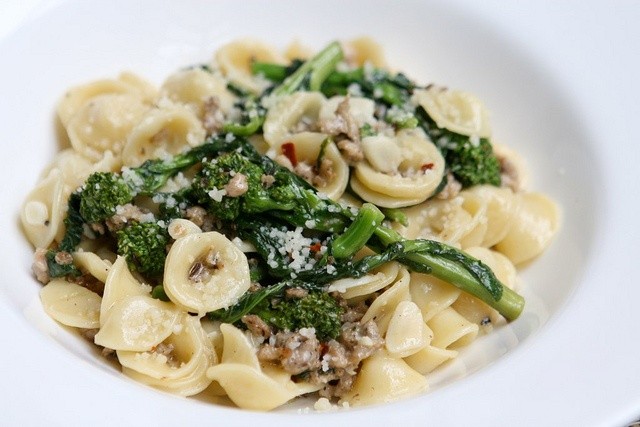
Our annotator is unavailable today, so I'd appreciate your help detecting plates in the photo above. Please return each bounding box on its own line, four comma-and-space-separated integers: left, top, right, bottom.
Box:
0, 1, 637, 427
